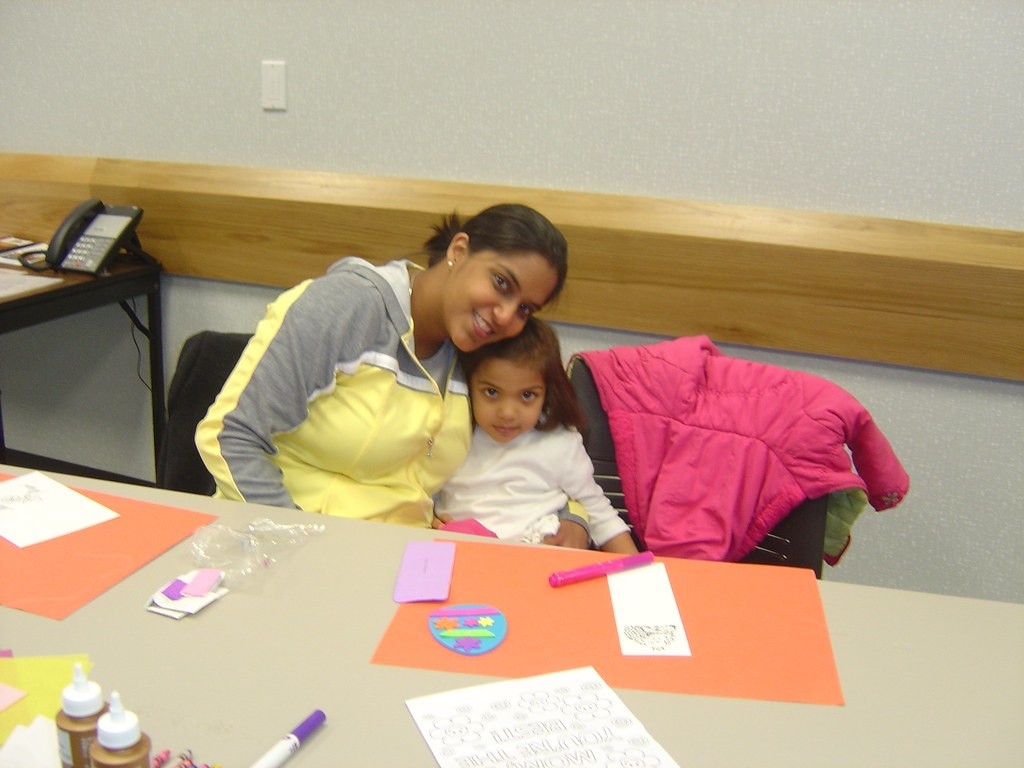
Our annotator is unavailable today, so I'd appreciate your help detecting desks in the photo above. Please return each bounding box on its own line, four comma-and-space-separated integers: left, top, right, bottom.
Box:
1, 226, 170, 486
1, 463, 1024, 765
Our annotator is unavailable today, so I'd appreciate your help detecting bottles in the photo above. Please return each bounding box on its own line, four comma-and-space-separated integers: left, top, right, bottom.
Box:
88, 692, 154, 767
53, 663, 119, 767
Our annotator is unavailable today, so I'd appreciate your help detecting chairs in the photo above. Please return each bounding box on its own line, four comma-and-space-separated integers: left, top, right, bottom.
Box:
558, 348, 833, 585
158, 327, 265, 496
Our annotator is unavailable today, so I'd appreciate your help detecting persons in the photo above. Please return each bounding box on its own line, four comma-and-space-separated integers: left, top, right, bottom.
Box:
423, 314, 643, 559
194, 203, 592, 558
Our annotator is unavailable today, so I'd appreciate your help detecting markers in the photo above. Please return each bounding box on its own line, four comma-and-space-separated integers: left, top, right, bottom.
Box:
548, 551, 656, 590
254, 708, 326, 767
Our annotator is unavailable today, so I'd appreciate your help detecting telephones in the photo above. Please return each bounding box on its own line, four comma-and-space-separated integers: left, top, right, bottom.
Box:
44, 197, 144, 278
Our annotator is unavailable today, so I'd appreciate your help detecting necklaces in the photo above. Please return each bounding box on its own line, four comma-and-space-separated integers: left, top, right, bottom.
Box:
409, 264, 420, 295
